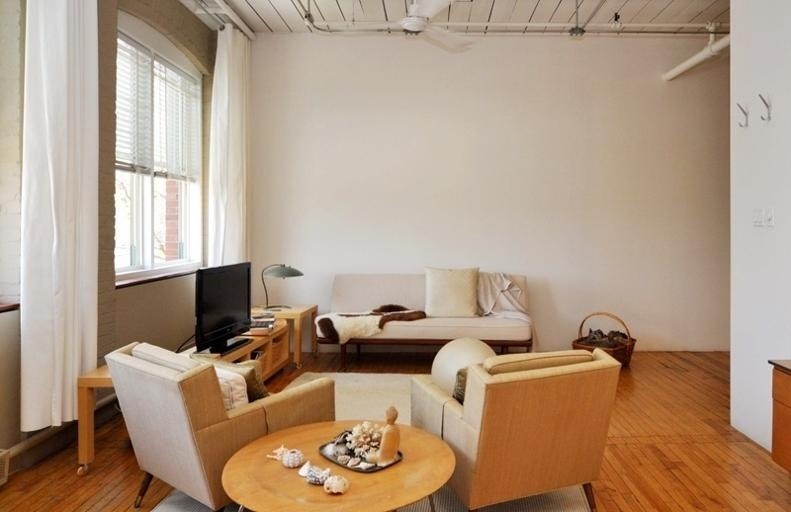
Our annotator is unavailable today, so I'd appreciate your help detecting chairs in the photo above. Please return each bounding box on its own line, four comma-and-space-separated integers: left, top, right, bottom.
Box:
104, 341, 335, 511
410, 347, 622, 511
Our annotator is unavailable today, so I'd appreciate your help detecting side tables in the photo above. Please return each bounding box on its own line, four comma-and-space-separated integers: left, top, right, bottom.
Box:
221, 419, 455, 510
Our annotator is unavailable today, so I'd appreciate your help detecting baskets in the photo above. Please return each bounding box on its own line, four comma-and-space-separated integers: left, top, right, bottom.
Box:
570, 311, 637, 367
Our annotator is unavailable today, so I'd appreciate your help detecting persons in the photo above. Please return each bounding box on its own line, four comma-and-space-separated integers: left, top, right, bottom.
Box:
365, 406, 400, 464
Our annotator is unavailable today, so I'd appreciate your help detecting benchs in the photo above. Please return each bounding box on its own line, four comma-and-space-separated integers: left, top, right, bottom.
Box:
315, 273, 532, 369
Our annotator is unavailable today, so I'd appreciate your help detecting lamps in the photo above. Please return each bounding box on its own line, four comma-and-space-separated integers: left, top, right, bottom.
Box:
261, 264, 303, 310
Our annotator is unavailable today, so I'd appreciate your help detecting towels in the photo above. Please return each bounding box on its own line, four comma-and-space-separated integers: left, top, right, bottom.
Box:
477, 271, 539, 351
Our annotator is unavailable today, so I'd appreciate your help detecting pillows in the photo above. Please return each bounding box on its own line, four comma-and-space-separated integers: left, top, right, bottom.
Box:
424, 267, 480, 318
452, 364, 476, 406
190, 354, 270, 401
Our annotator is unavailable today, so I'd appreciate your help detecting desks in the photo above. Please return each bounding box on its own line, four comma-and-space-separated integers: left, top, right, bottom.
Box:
77, 321, 290, 463
252, 305, 319, 369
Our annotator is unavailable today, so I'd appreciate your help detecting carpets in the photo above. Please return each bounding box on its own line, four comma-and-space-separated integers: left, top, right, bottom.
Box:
150, 371, 596, 512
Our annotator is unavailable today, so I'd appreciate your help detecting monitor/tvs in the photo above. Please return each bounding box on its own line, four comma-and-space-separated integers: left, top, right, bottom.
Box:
195, 262, 251, 355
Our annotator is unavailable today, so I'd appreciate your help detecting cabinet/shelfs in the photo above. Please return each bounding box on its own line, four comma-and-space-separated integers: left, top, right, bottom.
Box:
769, 360, 791, 471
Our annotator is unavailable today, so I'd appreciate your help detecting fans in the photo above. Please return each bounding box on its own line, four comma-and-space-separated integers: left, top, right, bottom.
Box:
313, 0, 473, 57
